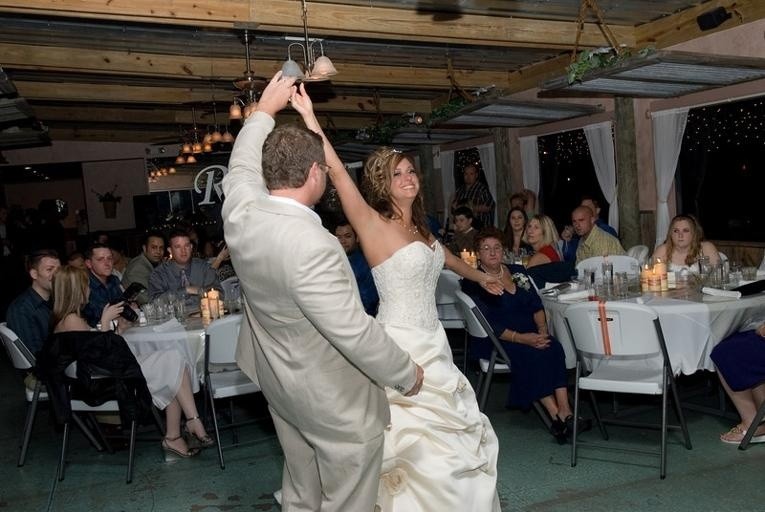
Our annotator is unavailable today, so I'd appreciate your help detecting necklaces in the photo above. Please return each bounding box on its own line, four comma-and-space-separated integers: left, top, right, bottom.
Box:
396, 219, 421, 235
480, 262, 505, 280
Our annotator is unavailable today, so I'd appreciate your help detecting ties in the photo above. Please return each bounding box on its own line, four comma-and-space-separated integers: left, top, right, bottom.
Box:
181, 269, 191, 287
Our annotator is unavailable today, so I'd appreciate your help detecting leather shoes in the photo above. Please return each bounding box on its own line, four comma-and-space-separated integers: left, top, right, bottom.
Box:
551, 414, 593, 444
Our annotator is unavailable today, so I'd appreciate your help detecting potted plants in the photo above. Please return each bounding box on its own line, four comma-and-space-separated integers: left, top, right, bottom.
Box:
324, 1, 765, 162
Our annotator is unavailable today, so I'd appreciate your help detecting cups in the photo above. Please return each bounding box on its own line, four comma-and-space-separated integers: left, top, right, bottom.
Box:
583, 262, 641, 298
674, 254, 758, 290
502, 247, 530, 266
139, 294, 185, 327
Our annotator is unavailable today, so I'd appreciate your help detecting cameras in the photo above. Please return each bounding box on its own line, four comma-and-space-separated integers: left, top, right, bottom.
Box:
112, 283, 145, 322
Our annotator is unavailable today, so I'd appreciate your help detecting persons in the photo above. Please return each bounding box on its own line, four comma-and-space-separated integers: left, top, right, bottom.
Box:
11, 231, 236, 378
46, 260, 219, 462
451, 228, 591, 446
214, 64, 428, 512
447, 162, 495, 228
284, 79, 508, 510
713, 318, 764, 446
424, 188, 723, 268
335, 214, 380, 319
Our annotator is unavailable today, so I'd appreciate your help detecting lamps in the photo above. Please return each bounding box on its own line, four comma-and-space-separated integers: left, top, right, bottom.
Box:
278, 1, 339, 83
146, 32, 270, 184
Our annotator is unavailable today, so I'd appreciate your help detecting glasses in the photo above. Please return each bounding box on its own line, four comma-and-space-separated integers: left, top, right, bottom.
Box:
481, 245, 503, 252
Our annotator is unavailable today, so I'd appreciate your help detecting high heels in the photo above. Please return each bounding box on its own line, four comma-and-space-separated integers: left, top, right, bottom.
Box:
163, 416, 216, 462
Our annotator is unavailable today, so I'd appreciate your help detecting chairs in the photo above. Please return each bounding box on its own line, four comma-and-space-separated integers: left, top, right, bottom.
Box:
627, 244, 649, 261
437, 269, 472, 379
32, 328, 166, 487
199, 312, 277, 471
0, 320, 104, 469
562, 301, 692, 480
452, 289, 557, 439
577, 253, 640, 278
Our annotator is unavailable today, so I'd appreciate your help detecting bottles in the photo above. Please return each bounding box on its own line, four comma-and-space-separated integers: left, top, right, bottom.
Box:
199, 286, 224, 318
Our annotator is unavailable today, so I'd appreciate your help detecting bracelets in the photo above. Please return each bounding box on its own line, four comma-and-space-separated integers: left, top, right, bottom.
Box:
477, 272, 493, 288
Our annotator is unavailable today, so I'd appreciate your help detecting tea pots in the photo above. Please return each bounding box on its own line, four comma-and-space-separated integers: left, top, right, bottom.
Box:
221, 277, 242, 314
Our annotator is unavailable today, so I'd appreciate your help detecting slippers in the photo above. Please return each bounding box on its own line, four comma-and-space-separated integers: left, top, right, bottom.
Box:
720, 422, 765, 444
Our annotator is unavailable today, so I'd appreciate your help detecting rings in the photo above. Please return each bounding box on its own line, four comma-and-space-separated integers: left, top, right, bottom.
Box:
286, 96, 292, 103
